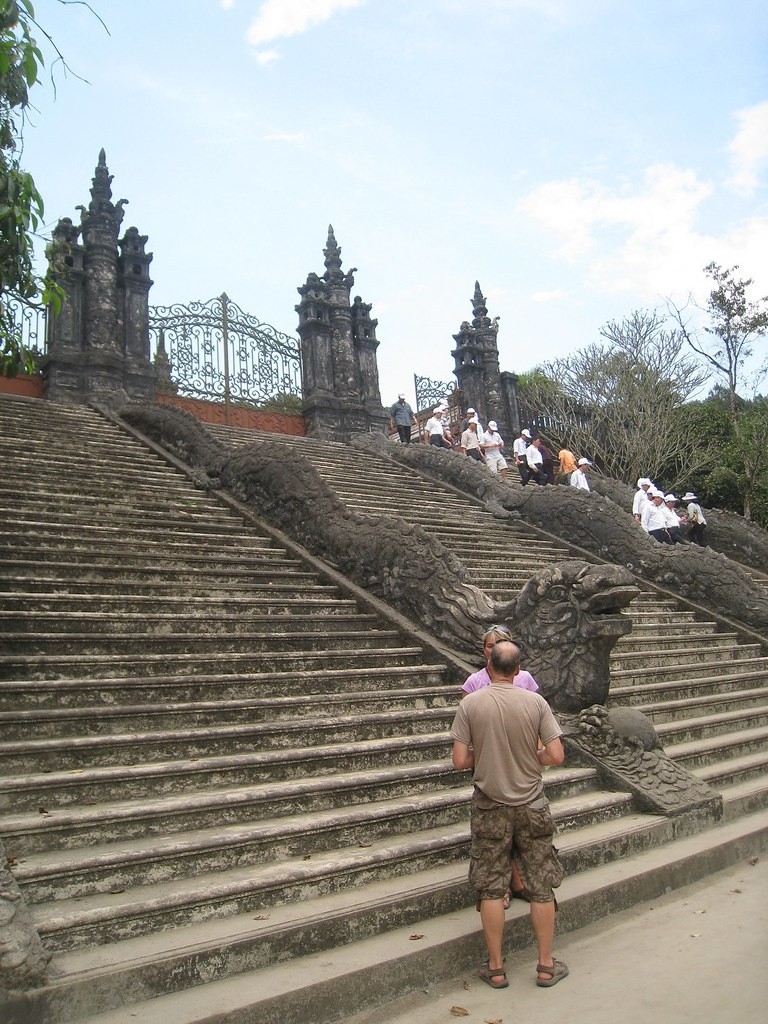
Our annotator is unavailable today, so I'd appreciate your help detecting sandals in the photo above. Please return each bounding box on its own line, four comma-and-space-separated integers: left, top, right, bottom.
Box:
536, 956, 569, 986
478, 962, 509, 989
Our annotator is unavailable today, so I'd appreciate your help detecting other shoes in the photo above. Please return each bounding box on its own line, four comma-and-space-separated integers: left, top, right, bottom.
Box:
502, 887, 532, 909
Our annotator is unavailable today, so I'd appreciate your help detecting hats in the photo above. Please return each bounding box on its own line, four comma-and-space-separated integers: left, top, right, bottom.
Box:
469, 418, 480, 425
438, 405, 450, 411
521, 429, 532, 439
665, 494, 678, 502
641, 478, 668, 501
398, 393, 406, 399
578, 458, 592, 467
682, 492, 697, 500
433, 408, 445, 415
488, 420, 499, 431
467, 407, 475, 414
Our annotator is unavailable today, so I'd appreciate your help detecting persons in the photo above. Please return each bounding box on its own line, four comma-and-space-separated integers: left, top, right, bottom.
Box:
424, 404, 593, 492
462, 626, 542, 910
633, 478, 707, 546
389, 393, 417, 444
450, 639, 570, 989
